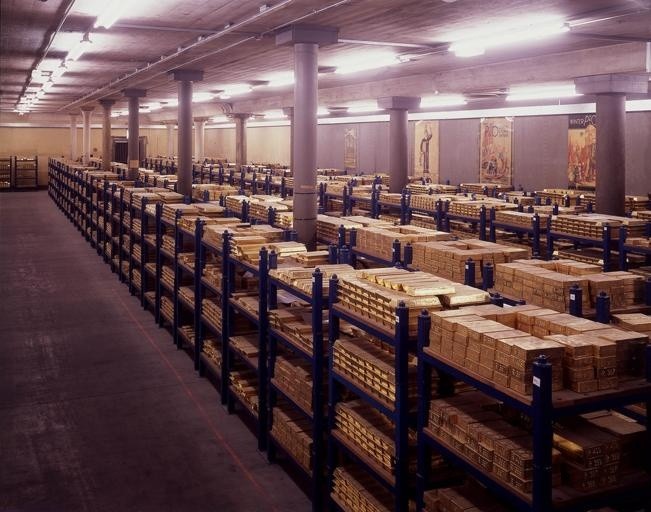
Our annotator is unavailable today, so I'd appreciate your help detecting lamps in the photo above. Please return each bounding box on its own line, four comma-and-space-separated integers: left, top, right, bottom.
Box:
26, 31, 93, 101
560, 22, 574, 34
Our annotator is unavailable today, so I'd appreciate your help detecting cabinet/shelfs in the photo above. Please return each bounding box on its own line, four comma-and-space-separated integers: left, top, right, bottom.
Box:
324, 244, 493, 511
258, 238, 402, 501
15, 155, 39, 192
414, 291, 651, 511
47, 153, 650, 370
195, 216, 293, 392
0, 155, 13, 192
223, 225, 348, 438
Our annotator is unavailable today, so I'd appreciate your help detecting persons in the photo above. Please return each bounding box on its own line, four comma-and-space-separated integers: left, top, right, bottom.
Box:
500, 147, 510, 174
420, 126, 433, 174
482, 125, 495, 168
487, 156, 499, 176
